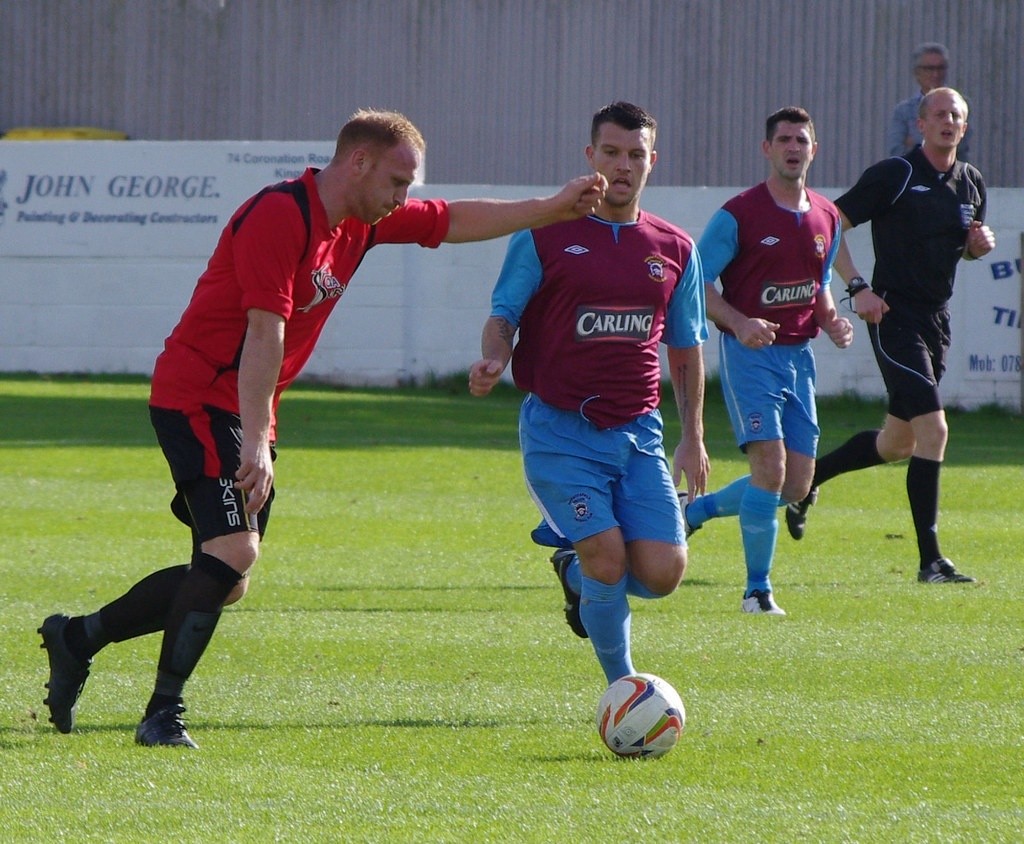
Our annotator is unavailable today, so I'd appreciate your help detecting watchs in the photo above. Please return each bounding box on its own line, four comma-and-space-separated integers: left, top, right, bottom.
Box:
847, 276, 865, 290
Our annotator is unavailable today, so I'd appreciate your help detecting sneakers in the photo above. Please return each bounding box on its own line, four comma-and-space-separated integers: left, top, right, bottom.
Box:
38, 614, 93, 734
742, 589, 786, 615
785, 486, 819, 540
135, 704, 199, 750
917, 557, 976, 583
675, 489, 702, 540
553, 549, 588, 639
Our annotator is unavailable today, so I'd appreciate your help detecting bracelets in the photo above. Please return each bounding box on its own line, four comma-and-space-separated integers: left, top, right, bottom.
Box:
845, 282, 869, 298
961, 242, 979, 261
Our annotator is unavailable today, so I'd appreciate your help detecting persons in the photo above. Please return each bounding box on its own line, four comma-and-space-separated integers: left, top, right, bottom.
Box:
884, 41, 974, 164
783, 85, 996, 584
467, 99, 712, 686
677, 105, 856, 616
37, 108, 611, 750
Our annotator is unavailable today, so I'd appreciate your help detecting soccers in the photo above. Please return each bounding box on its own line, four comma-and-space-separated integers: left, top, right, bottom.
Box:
595, 673, 686, 761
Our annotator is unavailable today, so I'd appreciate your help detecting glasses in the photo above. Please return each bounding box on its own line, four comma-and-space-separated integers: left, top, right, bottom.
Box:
915, 63, 948, 70
